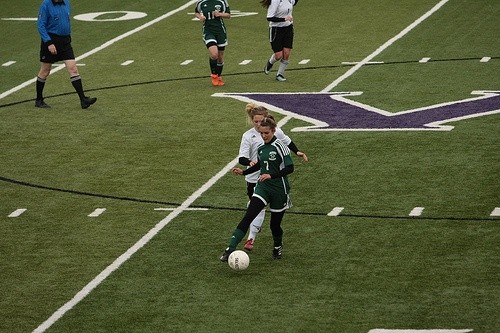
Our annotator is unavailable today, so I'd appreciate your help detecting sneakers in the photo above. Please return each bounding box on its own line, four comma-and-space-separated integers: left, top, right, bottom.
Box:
81, 96, 97, 110
211, 73, 219, 86
35, 98, 51, 108
276, 74, 286, 81
244, 239, 254, 250
217, 76, 224, 85
273, 242, 284, 259
219, 247, 234, 261
264, 58, 271, 74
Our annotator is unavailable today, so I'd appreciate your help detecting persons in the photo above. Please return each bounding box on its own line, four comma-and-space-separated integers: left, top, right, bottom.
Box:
35, 0, 97, 109
220, 113, 294, 263
260, 0, 299, 82
239, 102, 308, 251
194, 0, 232, 87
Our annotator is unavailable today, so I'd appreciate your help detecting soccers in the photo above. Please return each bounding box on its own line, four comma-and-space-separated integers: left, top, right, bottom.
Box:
228, 250, 251, 272
